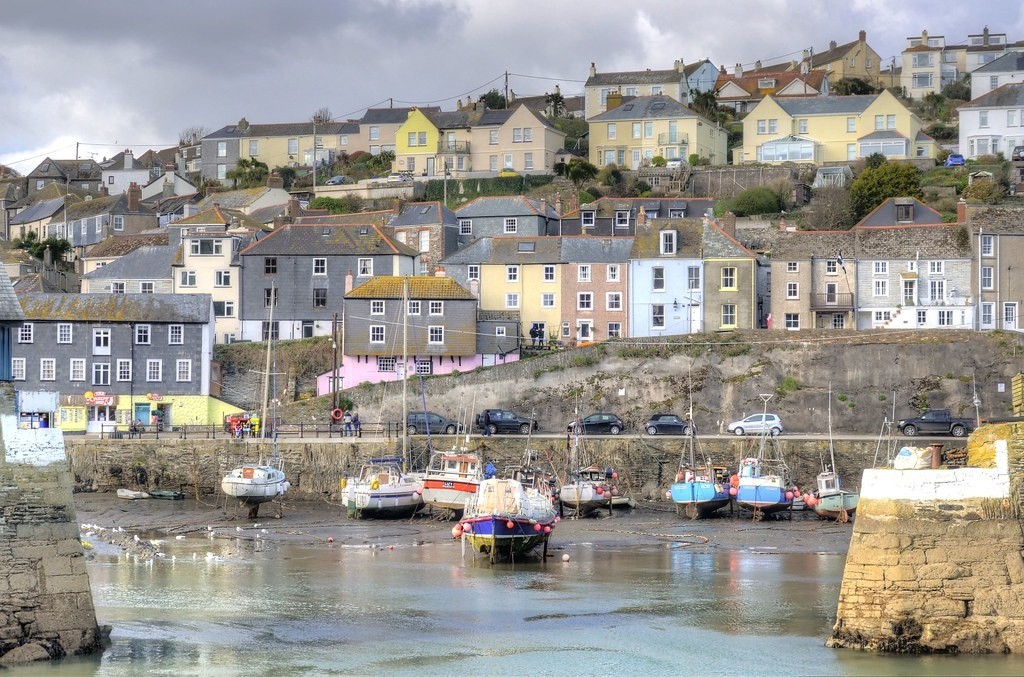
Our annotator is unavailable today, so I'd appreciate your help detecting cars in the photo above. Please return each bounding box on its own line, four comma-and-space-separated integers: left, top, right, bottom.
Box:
644, 414, 699, 437
566, 412, 625, 435
401, 411, 464, 435
726, 413, 784, 437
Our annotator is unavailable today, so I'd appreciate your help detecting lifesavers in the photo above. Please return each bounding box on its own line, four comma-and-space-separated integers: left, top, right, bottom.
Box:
372, 480, 379, 490
333, 408, 343, 420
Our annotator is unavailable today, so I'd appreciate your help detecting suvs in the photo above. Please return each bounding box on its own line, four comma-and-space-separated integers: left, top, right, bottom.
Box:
475, 409, 539, 435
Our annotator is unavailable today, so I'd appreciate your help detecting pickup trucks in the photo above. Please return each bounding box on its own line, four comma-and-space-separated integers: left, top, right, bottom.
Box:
896, 409, 974, 438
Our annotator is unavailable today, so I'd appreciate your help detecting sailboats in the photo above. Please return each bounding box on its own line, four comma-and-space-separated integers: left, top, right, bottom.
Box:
340, 274, 433, 519
220, 279, 291, 519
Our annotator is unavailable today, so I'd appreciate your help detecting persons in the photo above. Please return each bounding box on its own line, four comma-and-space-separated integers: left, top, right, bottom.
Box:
131, 419, 141, 435
530, 326, 543, 346
235, 420, 242, 438
482, 410, 491, 436
344, 410, 358, 436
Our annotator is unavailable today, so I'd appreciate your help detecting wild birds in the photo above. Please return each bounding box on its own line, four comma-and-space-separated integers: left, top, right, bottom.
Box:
79, 523, 269, 564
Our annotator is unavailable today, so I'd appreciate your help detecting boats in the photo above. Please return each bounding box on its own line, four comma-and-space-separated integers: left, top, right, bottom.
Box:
116, 488, 150, 500
419, 389, 497, 521
559, 391, 618, 518
730, 393, 801, 521
451, 418, 559, 564
604, 496, 631, 507
807, 380, 861, 525
149, 489, 186, 501
665, 362, 731, 521
